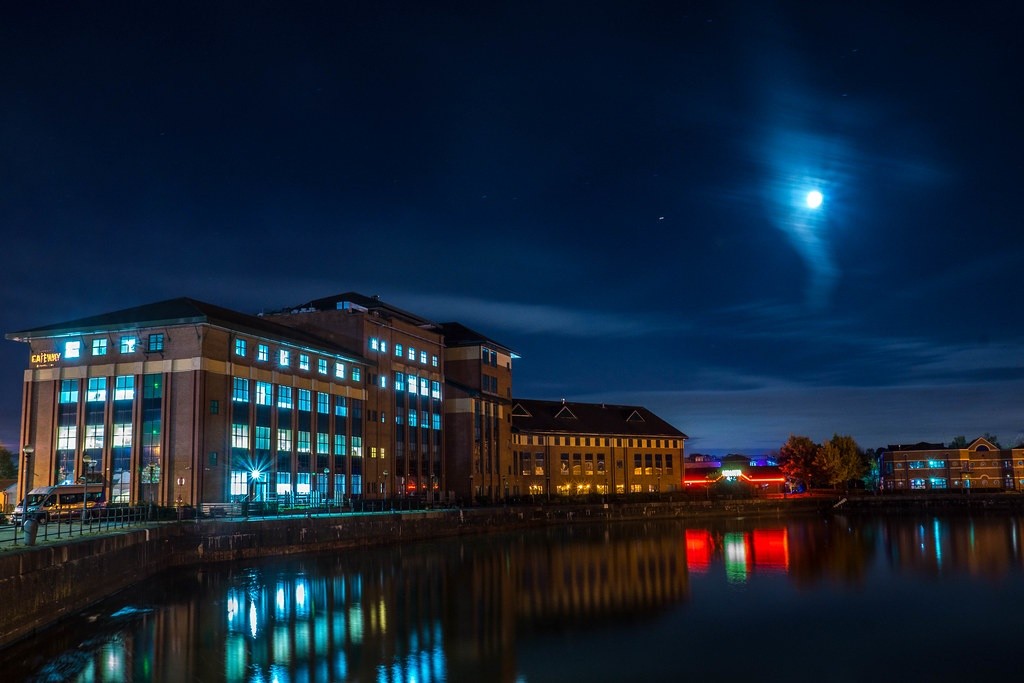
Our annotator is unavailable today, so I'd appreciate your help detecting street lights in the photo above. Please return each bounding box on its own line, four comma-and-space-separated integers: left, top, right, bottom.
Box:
807, 472, 812, 496
20, 440, 34, 530
430, 472, 436, 510
382, 469, 388, 510
324, 467, 330, 513
252, 469, 260, 503
148, 461, 156, 520
469, 474, 473, 508
88, 458, 98, 483
706, 475, 709, 500
81, 453, 93, 524
546, 474, 550, 501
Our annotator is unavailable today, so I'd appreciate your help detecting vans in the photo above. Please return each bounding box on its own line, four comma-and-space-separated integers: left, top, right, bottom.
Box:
10, 483, 103, 525
